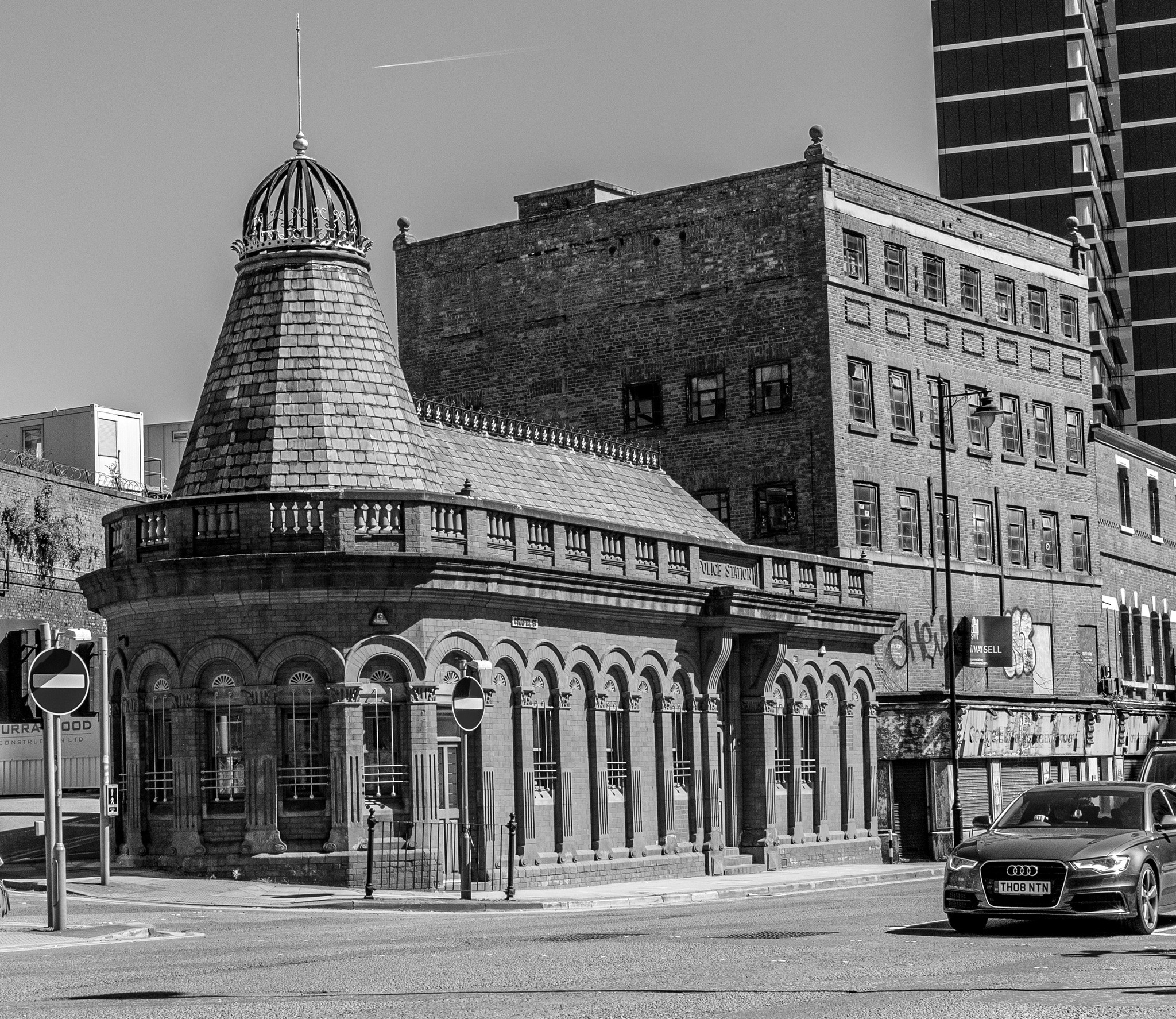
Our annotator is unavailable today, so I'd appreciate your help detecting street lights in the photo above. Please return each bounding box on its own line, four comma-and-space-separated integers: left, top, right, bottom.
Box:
928, 372, 1011, 845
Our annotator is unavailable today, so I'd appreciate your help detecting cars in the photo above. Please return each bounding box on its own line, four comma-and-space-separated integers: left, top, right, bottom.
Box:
942, 780, 1176, 936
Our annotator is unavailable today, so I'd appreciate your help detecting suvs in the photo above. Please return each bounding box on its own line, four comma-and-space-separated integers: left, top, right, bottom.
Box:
1133, 740, 1176, 811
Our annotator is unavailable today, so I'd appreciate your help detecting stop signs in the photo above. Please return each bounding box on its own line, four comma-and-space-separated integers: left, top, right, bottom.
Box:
27, 646, 90, 716
451, 675, 486, 731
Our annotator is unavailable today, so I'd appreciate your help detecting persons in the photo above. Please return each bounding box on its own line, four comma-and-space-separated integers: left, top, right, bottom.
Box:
1033, 796, 1079, 822
